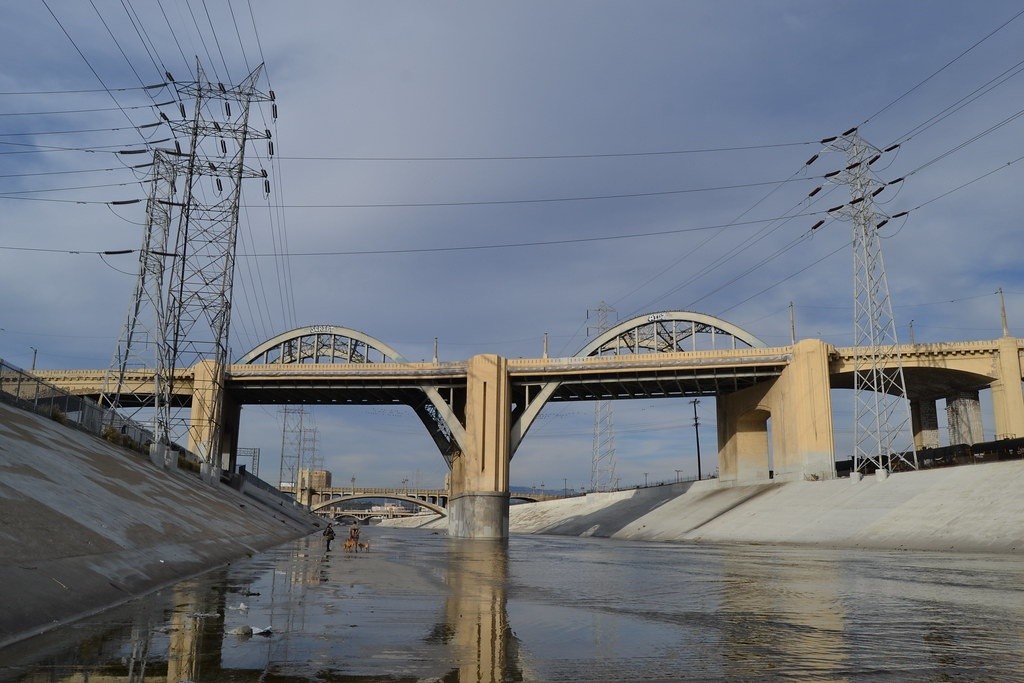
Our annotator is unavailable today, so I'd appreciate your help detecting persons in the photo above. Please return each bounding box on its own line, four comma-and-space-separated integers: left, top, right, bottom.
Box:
350, 521, 360, 553
323, 523, 336, 551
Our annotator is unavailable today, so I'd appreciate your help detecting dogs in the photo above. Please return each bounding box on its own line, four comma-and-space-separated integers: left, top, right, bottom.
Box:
358, 542, 369, 553
